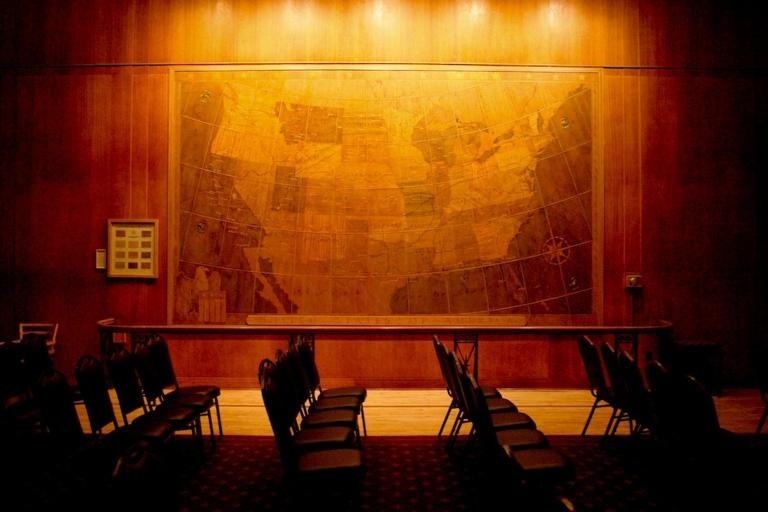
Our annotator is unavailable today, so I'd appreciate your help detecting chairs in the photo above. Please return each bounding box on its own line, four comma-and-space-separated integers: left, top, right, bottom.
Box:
260, 333, 374, 499
427, 334, 578, 510
576, 334, 723, 476
3, 328, 223, 510
